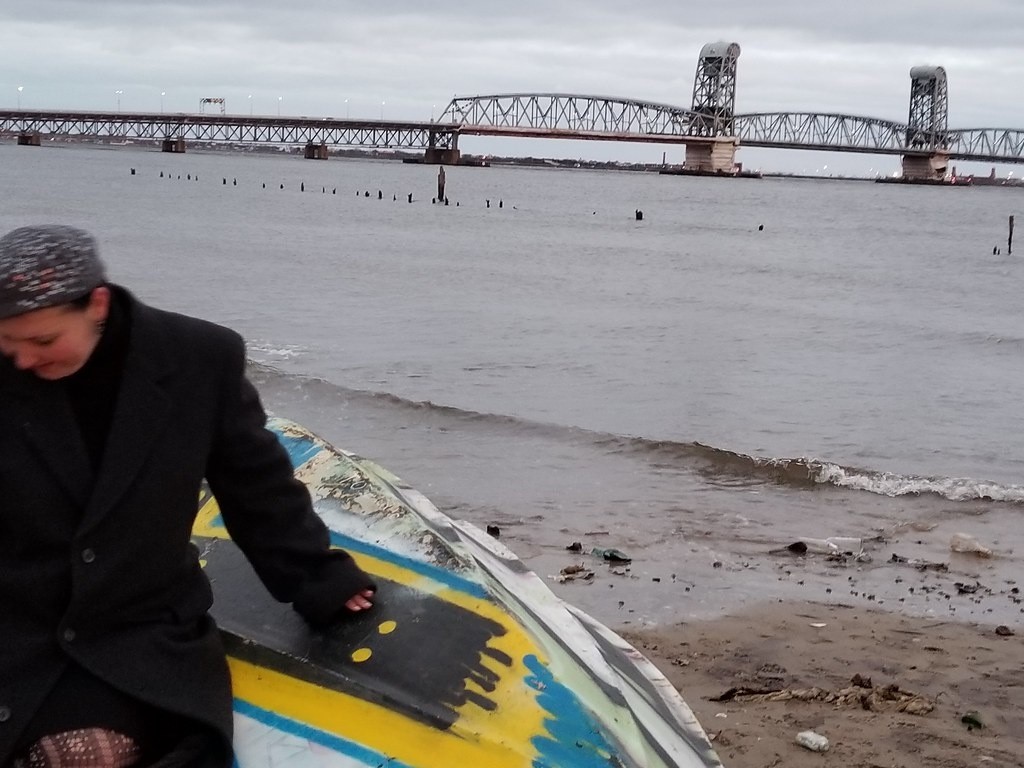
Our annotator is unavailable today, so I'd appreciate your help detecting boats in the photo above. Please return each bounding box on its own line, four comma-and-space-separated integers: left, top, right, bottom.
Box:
192, 412, 722, 768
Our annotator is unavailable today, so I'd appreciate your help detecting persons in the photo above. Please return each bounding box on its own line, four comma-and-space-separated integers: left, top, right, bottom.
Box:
0, 223, 375, 768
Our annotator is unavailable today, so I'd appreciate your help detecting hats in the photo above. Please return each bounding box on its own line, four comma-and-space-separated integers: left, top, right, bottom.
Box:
0, 224, 102, 318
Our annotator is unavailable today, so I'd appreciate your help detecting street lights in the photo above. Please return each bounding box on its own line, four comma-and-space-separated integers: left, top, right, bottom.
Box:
248, 94, 253, 116
116, 91, 122, 115
381, 101, 385, 120
161, 92, 166, 113
433, 104, 436, 122
279, 96, 282, 120
345, 99, 349, 121
18, 87, 24, 111
